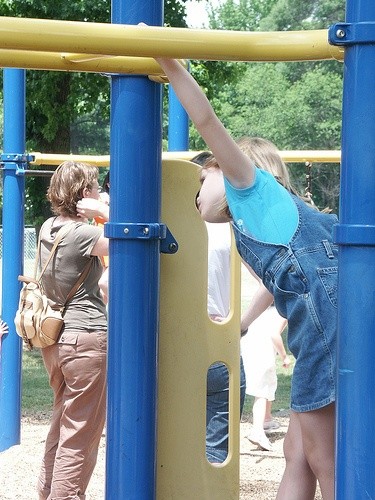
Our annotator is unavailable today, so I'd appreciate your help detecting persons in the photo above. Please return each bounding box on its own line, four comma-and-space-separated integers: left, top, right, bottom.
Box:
188, 153, 278, 463
134, 20, 341, 499
34, 158, 112, 500
0, 319, 8, 343
240, 293, 293, 452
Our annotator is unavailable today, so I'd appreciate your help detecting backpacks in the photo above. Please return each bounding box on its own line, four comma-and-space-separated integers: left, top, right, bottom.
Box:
15, 222, 94, 351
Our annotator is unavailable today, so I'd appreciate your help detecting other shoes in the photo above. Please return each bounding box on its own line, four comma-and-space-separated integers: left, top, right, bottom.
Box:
248, 429, 272, 450
264, 418, 281, 430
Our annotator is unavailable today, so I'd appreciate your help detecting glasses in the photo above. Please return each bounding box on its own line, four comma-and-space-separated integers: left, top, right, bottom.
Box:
84, 185, 103, 192
